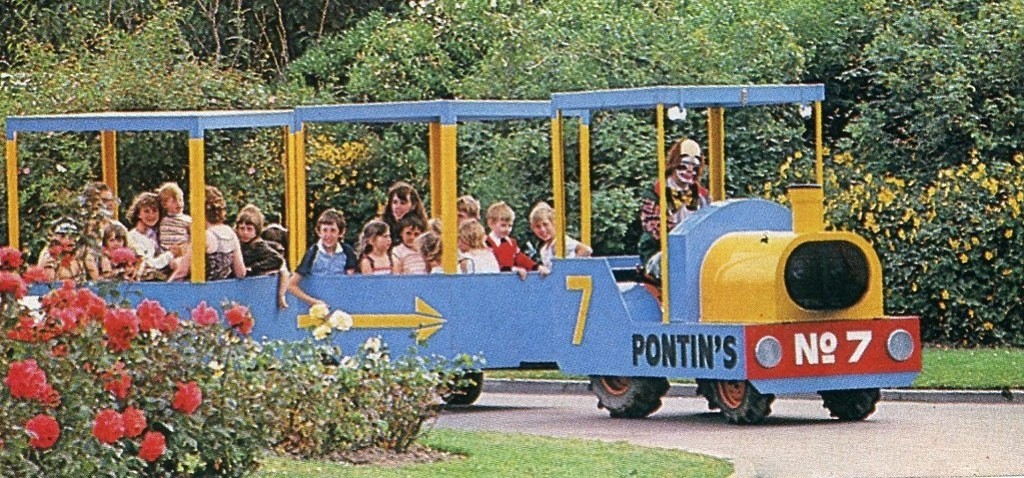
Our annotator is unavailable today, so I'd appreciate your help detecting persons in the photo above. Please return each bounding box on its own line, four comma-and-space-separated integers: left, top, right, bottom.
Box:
356, 180, 502, 276
637, 136, 711, 278
483, 199, 551, 280
289, 206, 359, 287
35, 181, 291, 310
528, 200, 593, 271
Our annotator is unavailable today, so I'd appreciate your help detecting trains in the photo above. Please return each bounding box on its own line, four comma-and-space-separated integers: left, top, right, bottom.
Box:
4, 82, 922, 426
